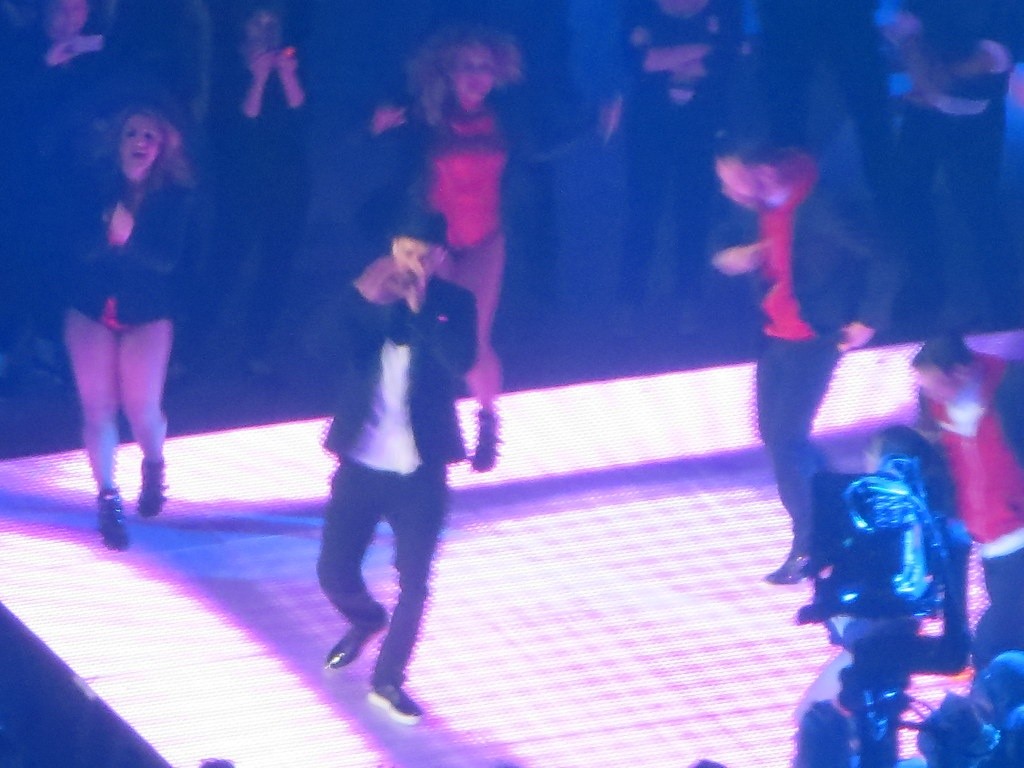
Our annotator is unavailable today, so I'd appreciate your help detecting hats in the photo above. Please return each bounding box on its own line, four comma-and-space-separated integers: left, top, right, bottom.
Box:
386, 201, 449, 250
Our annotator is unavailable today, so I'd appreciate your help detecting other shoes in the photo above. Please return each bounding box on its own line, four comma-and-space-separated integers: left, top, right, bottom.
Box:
97, 488, 130, 550
137, 457, 166, 517
368, 684, 424, 726
766, 551, 812, 584
327, 608, 388, 669
469, 410, 502, 471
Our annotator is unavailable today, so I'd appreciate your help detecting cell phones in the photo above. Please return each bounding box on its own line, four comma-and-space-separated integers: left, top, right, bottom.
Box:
71, 35, 104, 53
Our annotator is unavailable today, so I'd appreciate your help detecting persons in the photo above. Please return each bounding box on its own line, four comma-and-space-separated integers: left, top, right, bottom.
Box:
705, 136, 900, 584
369, 21, 527, 472
317, 210, 480, 723
792, 333, 1024, 768
64, 105, 196, 550
1, 0, 1024, 432
